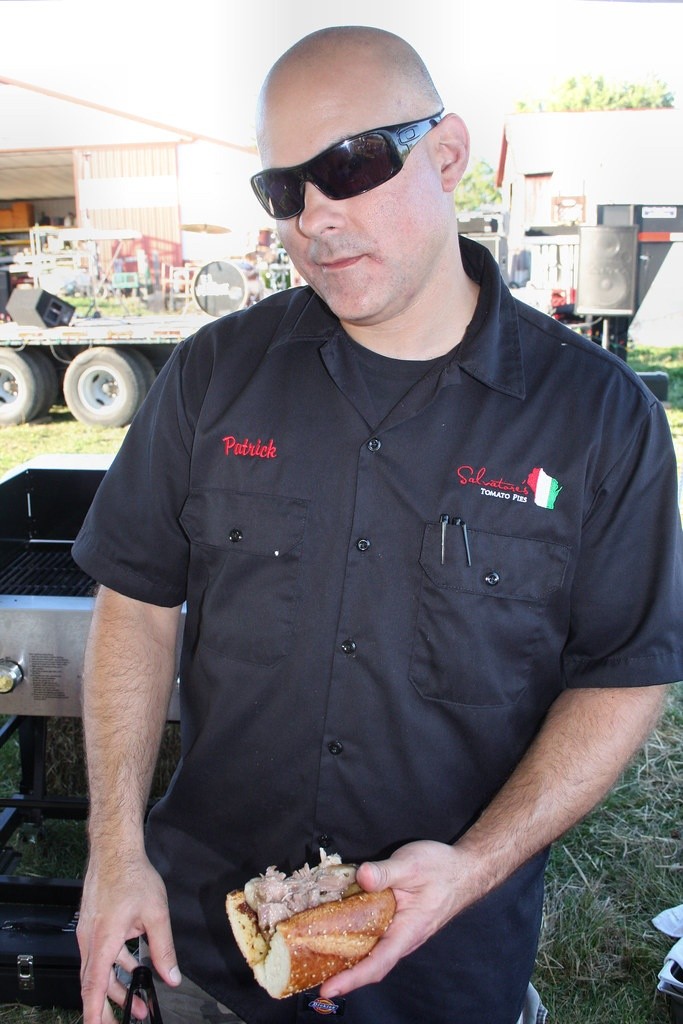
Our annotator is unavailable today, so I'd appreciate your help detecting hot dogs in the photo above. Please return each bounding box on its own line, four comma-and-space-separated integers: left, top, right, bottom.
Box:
225, 847, 396, 999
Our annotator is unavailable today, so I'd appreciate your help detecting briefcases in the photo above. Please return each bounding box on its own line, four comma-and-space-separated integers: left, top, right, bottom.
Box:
0, 877, 87, 1010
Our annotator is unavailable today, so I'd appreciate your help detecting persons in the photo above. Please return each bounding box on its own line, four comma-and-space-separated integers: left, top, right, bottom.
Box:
71, 25, 683, 1024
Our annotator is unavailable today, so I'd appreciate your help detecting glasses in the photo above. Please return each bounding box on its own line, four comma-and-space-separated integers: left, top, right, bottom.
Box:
250, 108, 446, 221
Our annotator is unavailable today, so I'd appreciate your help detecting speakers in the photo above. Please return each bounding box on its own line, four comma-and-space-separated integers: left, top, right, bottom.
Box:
576, 225, 639, 316
469, 237, 508, 285
5, 288, 76, 328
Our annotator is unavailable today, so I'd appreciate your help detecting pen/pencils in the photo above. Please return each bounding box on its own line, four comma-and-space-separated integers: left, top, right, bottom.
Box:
437, 513, 473, 568
